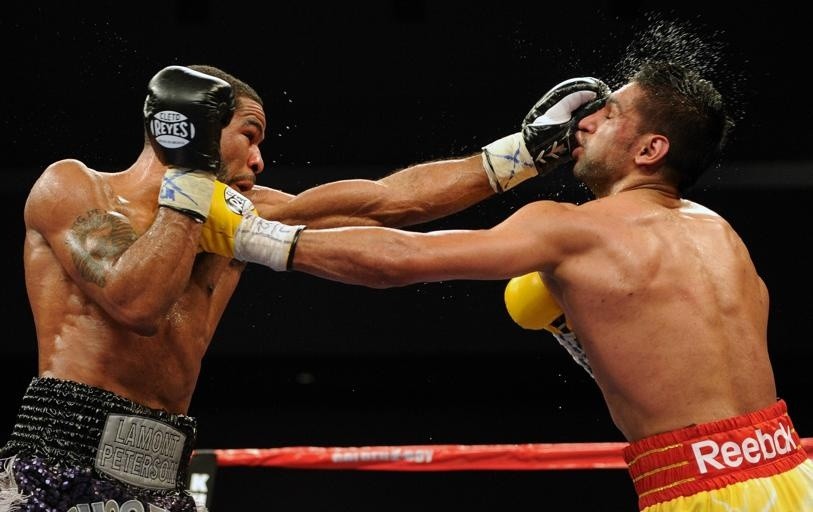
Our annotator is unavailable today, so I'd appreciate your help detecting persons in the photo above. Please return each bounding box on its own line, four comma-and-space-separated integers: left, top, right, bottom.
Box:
191, 61, 813, 511
0, 62, 613, 511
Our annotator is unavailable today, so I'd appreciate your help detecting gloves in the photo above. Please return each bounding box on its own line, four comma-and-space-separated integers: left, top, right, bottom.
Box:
144, 64, 236, 222
196, 180, 305, 273
505, 272, 594, 377
483, 76, 611, 195
